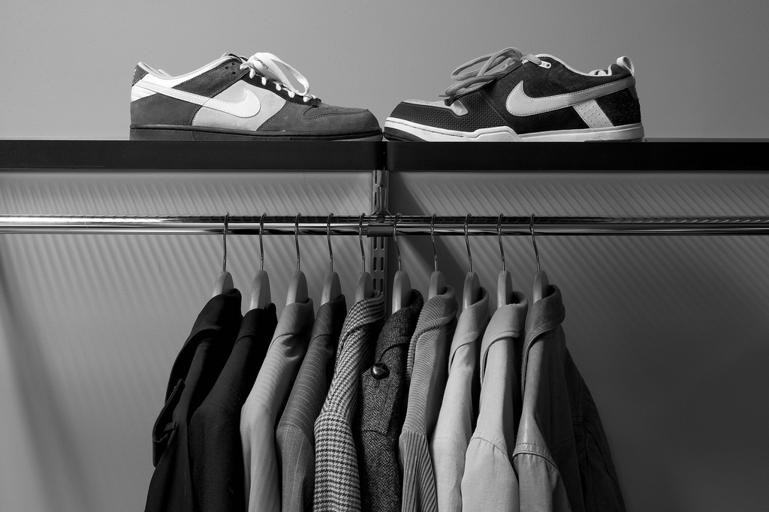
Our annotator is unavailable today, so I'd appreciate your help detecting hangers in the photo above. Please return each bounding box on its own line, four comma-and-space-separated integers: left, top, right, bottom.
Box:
211, 213, 548, 310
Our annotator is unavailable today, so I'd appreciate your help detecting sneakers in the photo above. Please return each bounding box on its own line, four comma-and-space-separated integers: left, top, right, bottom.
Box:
130, 51, 385, 140
385, 46, 644, 142
